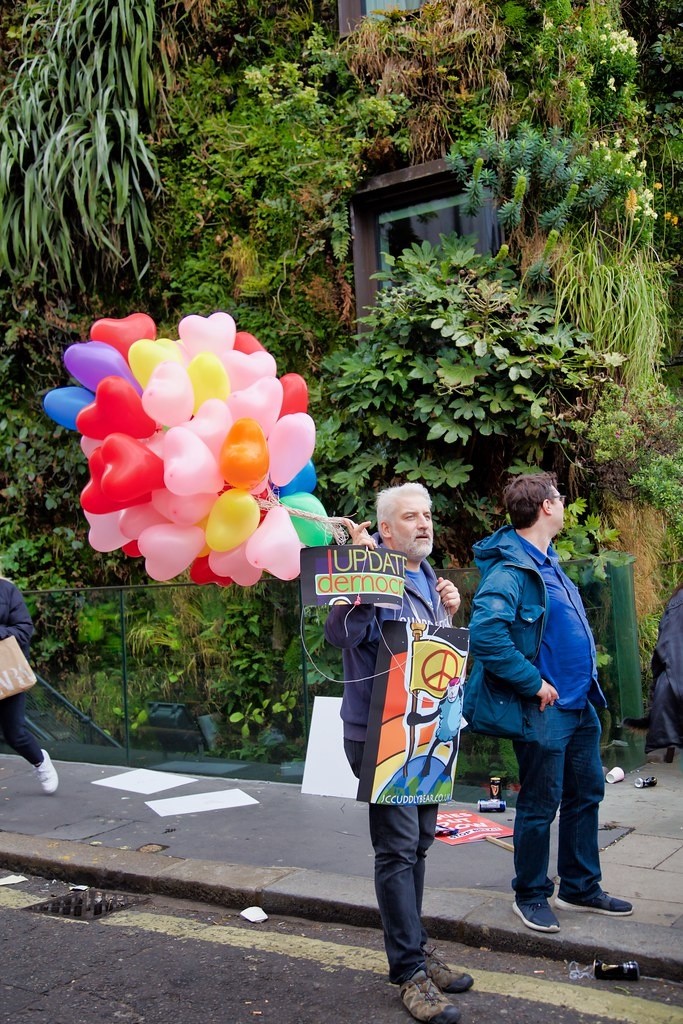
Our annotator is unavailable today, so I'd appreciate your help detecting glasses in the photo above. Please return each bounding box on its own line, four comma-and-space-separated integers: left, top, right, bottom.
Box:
540, 494, 567, 505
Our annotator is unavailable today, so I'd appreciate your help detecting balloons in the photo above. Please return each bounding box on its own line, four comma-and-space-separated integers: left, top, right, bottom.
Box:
44, 312, 332, 586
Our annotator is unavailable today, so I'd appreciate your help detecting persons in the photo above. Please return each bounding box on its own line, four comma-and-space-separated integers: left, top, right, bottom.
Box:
0, 577, 58, 793
326, 483, 473, 1024
624, 584, 683, 754
463, 473, 633, 931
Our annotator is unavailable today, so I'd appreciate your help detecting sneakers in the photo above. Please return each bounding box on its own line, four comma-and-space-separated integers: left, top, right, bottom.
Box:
511, 899, 559, 933
555, 886, 633, 916
422, 948, 474, 992
399, 965, 461, 1024
32, 747, 59, 793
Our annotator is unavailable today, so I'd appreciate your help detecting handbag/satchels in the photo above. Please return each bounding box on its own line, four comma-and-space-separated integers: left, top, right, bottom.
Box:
0, 635, 36, 700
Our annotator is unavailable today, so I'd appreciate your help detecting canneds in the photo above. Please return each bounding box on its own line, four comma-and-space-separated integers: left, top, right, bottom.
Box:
634, 776, 657, 788
490, 777, 501, 800
593, 958, 640, 981
477, 799, 506, 812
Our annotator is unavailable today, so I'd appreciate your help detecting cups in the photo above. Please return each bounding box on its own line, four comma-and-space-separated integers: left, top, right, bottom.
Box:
606, 767, 624, 783
